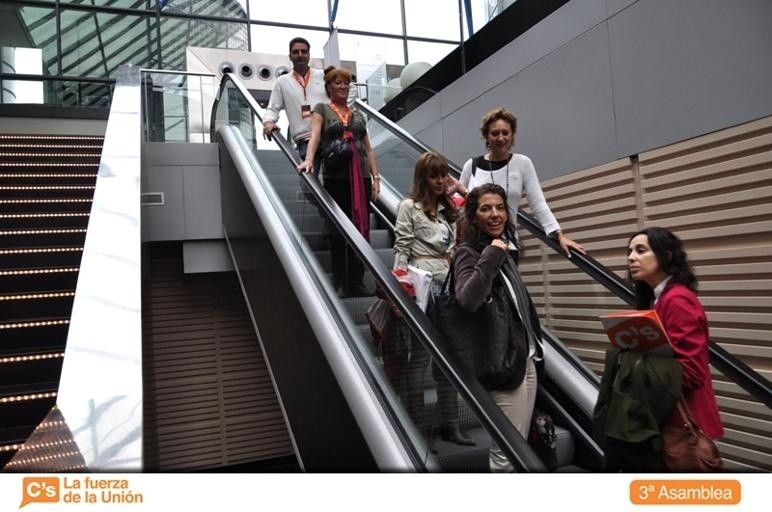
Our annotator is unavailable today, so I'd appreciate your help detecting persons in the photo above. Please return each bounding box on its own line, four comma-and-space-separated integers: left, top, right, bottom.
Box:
295, 67, 382, 299
364, 152, 479, 453
262, 38, 333, 251
453, 182, 545, 471
590, 224, 724, 471
459, 108, 587, 274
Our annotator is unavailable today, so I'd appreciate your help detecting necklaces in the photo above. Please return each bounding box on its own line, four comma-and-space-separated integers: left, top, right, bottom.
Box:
487, 149, 511, 202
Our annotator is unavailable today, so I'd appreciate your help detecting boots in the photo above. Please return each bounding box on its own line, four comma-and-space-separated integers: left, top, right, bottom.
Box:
436, 380, 477, 447
400, 391, 439, 454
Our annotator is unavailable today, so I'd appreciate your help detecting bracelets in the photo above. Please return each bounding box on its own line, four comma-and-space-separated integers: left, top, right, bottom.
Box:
554, 230, 562, 237
373, 175, 379, 182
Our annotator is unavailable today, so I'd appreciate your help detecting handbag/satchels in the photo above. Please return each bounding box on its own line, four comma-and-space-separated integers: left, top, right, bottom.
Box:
382, 316, 414, 393
427, 286, 517, 383
322, 141, 353, 176
660, 422, 722, 471
529, 410, 558, 471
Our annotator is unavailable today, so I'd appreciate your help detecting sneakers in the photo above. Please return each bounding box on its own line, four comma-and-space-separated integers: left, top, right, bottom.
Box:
335, 281, 345, 297
348, 281, 375, 297
